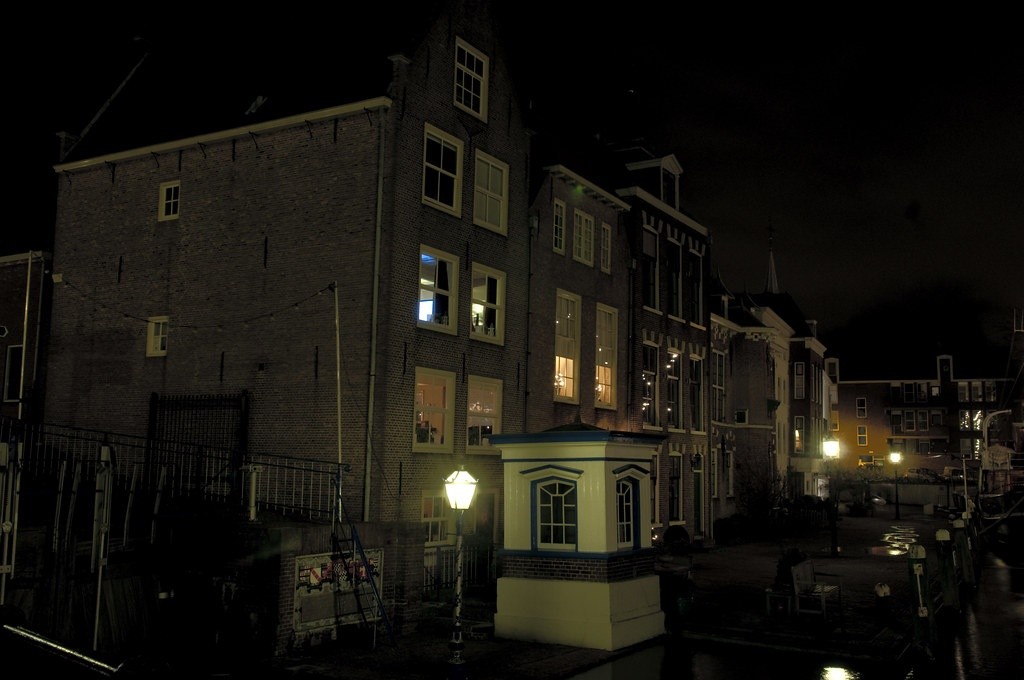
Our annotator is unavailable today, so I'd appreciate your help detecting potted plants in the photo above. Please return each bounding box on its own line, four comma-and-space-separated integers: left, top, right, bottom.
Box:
777, 548, 809, 606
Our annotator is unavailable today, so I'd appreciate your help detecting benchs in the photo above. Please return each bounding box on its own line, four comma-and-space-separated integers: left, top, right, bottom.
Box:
766, 592, 795, 616
794, 564, 842, 618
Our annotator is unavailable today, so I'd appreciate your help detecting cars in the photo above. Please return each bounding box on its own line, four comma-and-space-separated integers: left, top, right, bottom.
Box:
903, 467, 942, 484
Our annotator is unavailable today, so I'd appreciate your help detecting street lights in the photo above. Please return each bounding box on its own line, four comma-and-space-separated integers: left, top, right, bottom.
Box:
890, 448, 904, 519
442, 466, 480, 680
822, 427, 841, 554
